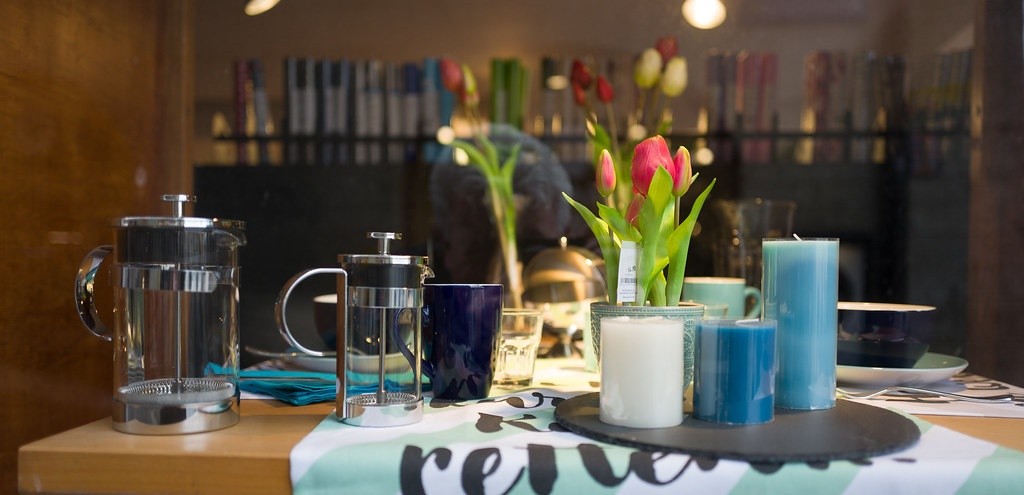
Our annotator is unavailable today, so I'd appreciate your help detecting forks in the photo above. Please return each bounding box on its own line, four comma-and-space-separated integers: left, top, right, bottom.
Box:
835, 386, 1015, 404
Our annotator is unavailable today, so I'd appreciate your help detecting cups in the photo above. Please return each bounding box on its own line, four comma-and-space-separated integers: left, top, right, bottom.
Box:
389, 284, 503, 401
491, 308, 546, 391
680, 277, 761, 319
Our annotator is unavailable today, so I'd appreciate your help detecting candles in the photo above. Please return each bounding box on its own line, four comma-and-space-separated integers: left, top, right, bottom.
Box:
598, 315, 685, 428
692, 313, 776, 426
761, 237, 839, 410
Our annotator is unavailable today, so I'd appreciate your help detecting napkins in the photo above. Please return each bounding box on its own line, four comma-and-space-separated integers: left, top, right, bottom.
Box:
204, 362, 429, 404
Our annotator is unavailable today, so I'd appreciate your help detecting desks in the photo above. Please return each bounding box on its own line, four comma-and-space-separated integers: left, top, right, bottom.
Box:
18, 354, 1024, 495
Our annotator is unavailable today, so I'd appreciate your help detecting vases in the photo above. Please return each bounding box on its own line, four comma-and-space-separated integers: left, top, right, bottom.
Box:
589, 302, 704, 403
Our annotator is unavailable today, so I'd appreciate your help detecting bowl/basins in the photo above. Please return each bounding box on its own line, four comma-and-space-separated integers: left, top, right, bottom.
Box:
837, 302, 937, 368
312, 294, 415, 354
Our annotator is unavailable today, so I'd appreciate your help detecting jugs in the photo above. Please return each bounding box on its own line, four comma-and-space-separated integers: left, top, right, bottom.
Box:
274, 228, 436, 430
73, 194, 247, 437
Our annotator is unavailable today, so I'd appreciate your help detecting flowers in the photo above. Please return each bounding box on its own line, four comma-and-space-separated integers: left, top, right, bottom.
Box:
567, 36, 716, 306
438, 58, 529, 328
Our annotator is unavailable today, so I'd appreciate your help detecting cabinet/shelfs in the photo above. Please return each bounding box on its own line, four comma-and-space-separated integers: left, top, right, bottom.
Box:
210, 131, 909, 329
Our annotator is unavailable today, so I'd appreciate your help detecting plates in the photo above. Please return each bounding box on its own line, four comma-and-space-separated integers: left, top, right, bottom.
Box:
836, 351, 970, 387
287, 349, 411, 373
